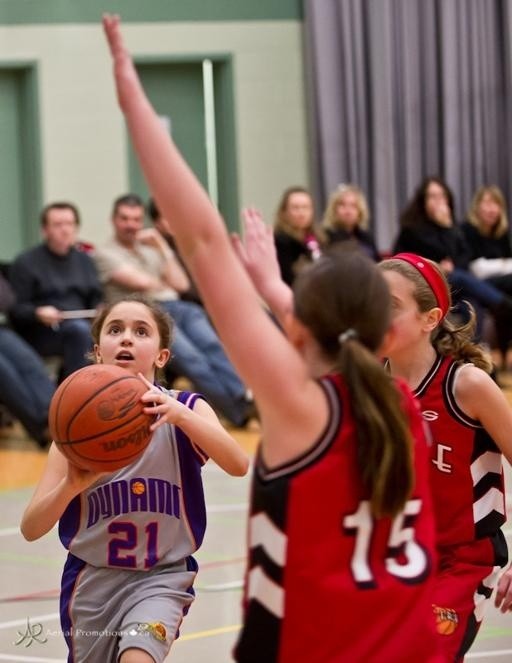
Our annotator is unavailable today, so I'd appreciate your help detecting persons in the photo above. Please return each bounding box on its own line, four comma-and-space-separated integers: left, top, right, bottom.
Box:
0, 173, 511, 462
102, 10, 439, 663
18, 292, 250, 662
228, 201, 511, 663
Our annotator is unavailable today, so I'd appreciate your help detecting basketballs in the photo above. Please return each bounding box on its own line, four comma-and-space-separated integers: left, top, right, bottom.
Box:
48, 364, 157, 473
